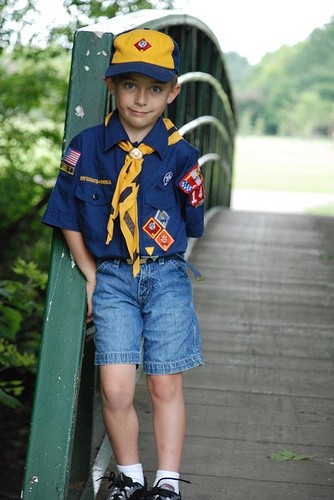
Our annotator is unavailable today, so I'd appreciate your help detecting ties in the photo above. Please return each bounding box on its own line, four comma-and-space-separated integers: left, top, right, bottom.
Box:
105, 112, 184, 278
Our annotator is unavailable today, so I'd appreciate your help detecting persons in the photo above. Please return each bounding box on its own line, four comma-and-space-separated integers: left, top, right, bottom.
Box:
41, 28, 204, 499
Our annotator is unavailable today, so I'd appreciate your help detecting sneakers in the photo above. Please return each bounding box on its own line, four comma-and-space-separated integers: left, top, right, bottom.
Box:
143, 478, 191, 500
96, 472, 147, 500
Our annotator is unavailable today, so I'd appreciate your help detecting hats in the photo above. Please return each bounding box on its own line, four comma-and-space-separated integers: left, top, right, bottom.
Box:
105, 28, 179, 81
